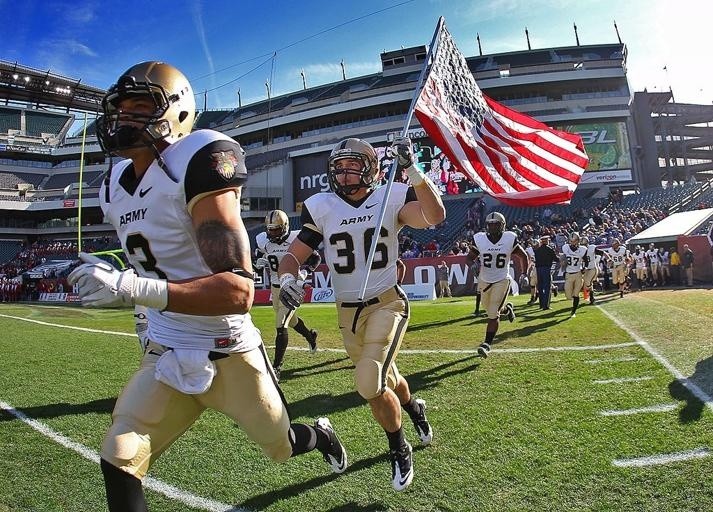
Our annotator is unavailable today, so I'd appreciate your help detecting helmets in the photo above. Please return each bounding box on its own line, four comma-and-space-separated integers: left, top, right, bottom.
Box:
264, 210, 289, 243
326, 137, 380, 195
485, 212, 688, 252
96, 61, 196, 157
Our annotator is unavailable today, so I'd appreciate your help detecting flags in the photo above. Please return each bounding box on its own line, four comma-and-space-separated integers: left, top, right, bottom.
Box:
412, 15, 590, 207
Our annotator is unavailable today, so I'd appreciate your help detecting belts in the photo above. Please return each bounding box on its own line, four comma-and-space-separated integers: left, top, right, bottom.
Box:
168, 347, 229, 361
341, 286, 398, 335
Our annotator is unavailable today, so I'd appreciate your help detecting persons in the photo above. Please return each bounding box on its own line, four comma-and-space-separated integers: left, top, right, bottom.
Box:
506, 200, 709, 304
276, 137, 448, 495
534, 236, 561, 310
63, 60, 346, 511
562, 232, 588, 317
472, 256, 484, 315
438, 260, 452, 298
398, 200, 489, 258
251, 209, 322, 374
466, 211, 515, 359
2, 235, 114, 304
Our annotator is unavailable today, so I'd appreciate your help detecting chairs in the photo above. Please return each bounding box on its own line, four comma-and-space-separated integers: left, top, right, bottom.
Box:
0, 45, 126, 267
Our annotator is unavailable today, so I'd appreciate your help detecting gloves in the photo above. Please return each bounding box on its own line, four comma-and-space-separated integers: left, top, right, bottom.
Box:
67, 252, 137, 308
279, 273, 306, 310
256, 258, 269, 270
391, 136, 414, 169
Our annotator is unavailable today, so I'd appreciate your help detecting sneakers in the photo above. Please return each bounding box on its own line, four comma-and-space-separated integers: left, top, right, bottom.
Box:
309, 329, 318, 353
389, 439, 414, 491
410, 399, 433, 443
273, 365, 281, 382
314, 417, 347, 473
475, 282, 693, 359
